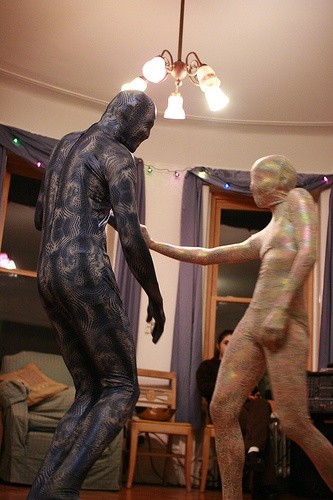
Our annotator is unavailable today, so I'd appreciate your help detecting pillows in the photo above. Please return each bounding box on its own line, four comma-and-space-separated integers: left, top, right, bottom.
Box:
0, 362, 70, 406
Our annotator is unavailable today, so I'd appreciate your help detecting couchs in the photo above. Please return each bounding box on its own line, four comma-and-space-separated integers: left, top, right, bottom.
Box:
2, 350, 124, 491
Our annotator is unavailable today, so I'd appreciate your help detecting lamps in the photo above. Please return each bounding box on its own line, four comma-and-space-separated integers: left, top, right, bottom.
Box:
121, 0, 230, 120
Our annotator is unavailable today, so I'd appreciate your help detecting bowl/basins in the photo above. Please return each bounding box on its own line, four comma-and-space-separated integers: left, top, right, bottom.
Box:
135, 406, 176, 423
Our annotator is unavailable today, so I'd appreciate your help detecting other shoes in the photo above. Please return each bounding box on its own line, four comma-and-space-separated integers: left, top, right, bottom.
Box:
245, 448, 264, 468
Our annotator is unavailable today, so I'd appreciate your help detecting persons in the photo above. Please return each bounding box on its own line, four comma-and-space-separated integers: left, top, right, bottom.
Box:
195, 329, 279, 487
26, 91, 165, 500
139, 155, 333, 498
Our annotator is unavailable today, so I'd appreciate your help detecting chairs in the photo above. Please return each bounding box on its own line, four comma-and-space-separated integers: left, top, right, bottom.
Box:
199, 396, 221, 495
123, 367, 196, 492
